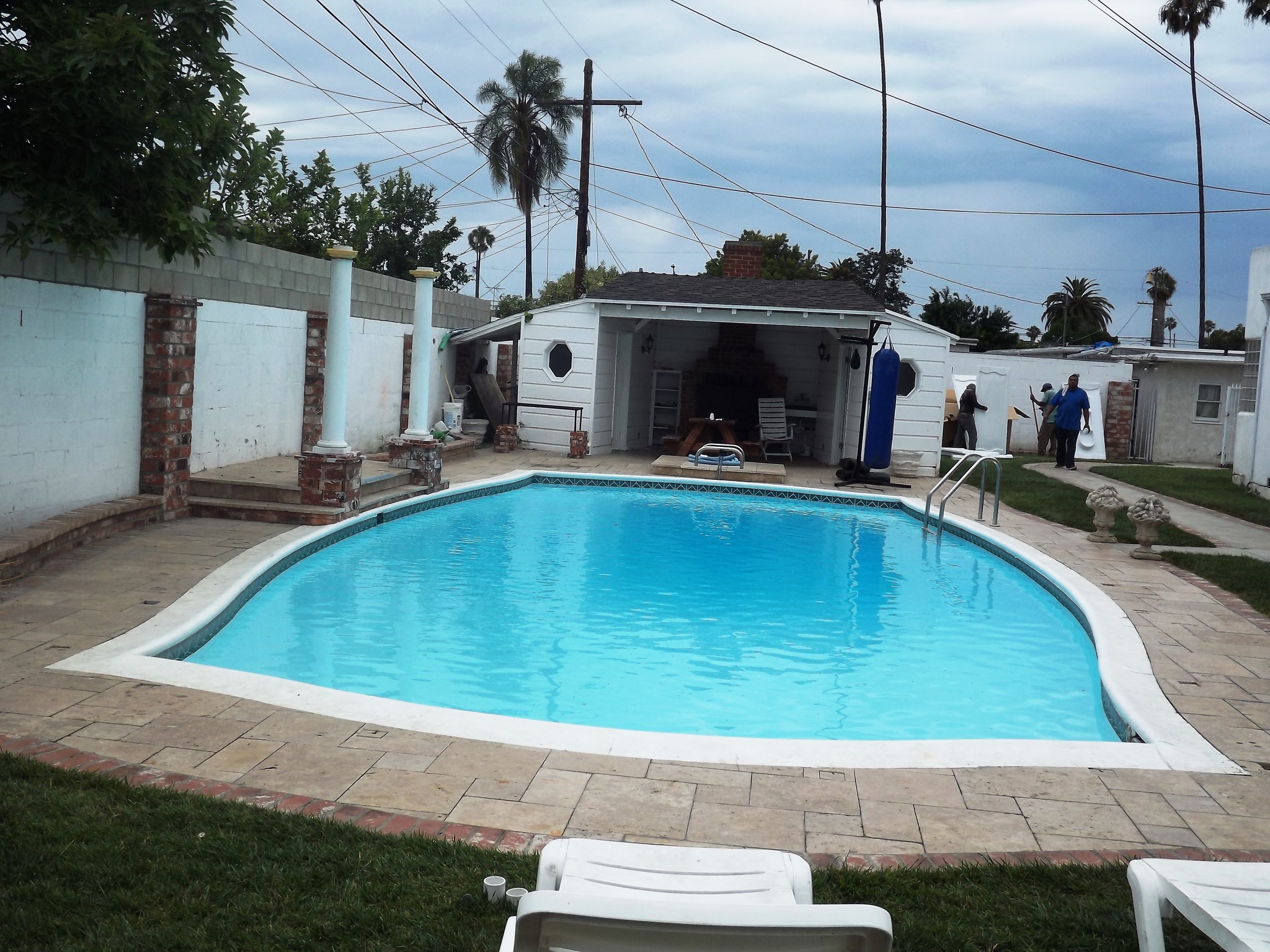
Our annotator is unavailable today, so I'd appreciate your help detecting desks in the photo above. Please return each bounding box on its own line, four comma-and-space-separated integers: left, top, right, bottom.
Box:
677, 416, 741, 461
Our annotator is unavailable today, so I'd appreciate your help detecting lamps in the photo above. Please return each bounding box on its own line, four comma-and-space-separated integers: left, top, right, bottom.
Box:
642, 332, 654, 353
1144, 361, 1159, 372
817, 340, 830, 362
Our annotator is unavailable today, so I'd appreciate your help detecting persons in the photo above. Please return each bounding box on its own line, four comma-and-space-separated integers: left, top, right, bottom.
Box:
1030, 383, 1058, 455
953, 384, 988, 451
1044, 375, 1090, 471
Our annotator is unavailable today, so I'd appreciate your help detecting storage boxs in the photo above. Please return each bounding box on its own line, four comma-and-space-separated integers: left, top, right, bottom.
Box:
942, 389, 1031, 454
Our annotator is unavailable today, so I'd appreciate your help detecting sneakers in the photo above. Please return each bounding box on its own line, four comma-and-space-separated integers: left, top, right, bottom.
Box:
1065, 466, 1077, 470
1054, 463, 1065, 468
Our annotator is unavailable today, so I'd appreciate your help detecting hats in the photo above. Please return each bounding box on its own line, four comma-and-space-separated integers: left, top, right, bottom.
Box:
1041, 383, 1052, 392
967, 383, 976, 391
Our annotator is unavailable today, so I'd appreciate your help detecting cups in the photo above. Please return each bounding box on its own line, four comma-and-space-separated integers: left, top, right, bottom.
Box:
1082, 429, 1088, 435
709, 413, 714, 420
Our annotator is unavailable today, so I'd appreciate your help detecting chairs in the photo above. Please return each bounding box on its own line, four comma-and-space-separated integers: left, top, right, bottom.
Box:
756, 398, 797, 462
499, 837, 894, 952
1127, 858, 1270, 952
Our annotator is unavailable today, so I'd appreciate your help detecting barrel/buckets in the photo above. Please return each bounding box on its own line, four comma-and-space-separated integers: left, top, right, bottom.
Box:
429, 428, 436, 434
461, 419, 489, 449
441, 402, 463, 433
892, 449, 924, 478
432, 421, 448, 431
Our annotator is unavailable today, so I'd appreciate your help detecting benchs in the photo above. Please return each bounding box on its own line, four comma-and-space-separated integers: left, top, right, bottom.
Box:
742, 436, 761, 456
662, 432, 688, 449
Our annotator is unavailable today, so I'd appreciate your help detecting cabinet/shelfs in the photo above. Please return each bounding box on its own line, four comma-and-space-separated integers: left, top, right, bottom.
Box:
649, 368, 683, 446
